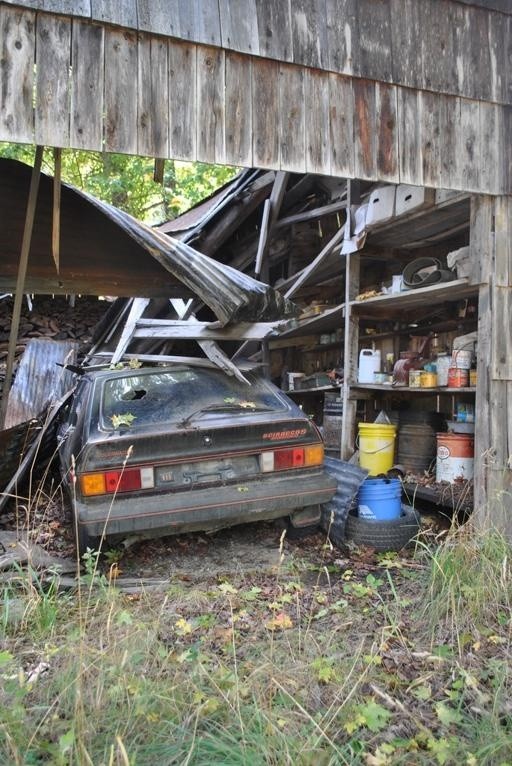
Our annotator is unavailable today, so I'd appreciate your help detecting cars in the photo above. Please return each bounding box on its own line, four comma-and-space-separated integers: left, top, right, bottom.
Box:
56, 367, 337, 546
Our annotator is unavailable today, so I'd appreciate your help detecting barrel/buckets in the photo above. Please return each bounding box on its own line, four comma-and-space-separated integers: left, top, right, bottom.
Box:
323, 392, 364, 460
398, 410, 445, 478
436, 432, 473, 483
356, 422, 397, 476
359, 348, 382, 383
356, 478, 402, 520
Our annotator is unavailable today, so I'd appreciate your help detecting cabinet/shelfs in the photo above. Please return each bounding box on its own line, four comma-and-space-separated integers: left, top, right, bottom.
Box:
266, 202, 347, 460
350, 195, 479, 514
